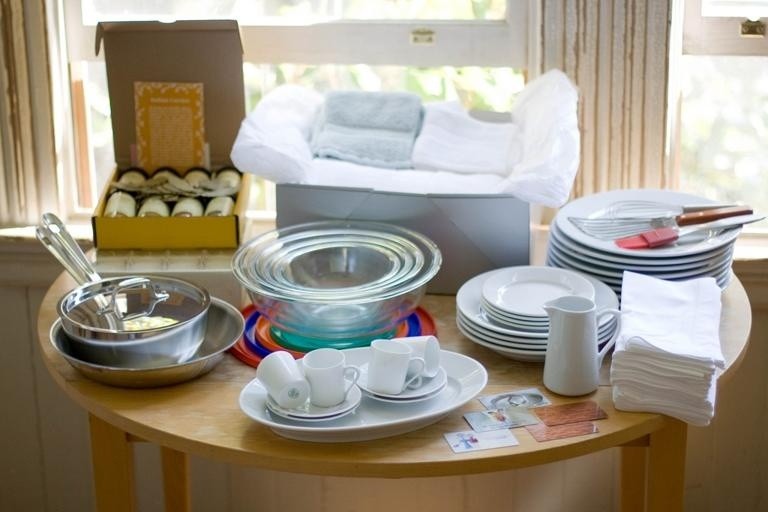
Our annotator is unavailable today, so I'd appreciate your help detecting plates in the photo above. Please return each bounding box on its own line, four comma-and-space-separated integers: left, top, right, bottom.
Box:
545, 189, 743, 310
238, 345, 497, 443
456, 266, 621, 366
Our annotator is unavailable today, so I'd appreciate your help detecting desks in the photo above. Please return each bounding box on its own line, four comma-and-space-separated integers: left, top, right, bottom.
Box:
35, 226, 755, 510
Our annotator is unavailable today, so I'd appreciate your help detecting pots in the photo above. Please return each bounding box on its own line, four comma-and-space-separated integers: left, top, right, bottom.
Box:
34, 207, 247, 393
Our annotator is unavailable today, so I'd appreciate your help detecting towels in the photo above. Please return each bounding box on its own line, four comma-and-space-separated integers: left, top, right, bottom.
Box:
609, 268, 726, 429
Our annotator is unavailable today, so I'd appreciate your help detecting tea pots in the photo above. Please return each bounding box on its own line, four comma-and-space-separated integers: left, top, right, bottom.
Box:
542, 292, 622, 399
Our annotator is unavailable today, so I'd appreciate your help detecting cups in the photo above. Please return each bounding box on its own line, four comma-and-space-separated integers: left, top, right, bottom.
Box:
103, 168, 241, 217
364, 333, 441, 394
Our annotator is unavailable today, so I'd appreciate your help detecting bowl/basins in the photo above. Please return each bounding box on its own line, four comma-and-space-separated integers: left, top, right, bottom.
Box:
231, 221, 443, 340
